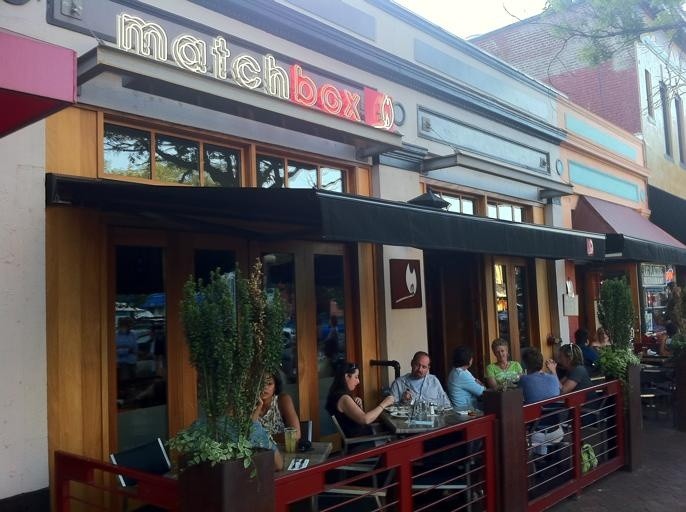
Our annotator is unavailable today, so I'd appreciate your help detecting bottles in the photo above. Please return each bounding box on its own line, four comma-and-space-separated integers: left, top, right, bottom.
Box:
414, 399, 438, 416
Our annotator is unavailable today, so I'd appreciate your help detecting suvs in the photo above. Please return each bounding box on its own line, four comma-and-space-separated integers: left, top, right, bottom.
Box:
116, 315, 166, 381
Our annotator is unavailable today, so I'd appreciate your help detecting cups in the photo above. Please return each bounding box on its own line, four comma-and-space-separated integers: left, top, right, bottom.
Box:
638, 347, 648, 358
497, 369, 528, 392
299, 420, 313, 443
284, 427, 296, 452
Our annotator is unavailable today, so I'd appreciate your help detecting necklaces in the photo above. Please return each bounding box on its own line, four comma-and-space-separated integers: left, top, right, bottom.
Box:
497, 360, 512, 373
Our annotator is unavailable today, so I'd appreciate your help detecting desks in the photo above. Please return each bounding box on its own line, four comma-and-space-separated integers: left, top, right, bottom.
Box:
153, 433, 333, 511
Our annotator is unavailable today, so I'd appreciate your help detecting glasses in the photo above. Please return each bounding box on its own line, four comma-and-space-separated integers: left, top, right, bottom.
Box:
351, 364, 359, 370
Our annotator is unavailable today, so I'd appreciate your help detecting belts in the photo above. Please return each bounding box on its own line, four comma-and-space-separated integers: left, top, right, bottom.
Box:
535, 425, 559, 433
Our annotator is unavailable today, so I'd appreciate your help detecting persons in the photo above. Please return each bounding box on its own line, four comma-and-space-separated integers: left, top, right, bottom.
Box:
642, 325, 682, 389
483, 338, 524, 391
387, 351, 452, 474
324, 362, 412, 486
245, 363, 303, 446
115, 309, 345, 407
592, 325, 613, 345
516, 348, 564, 469
171, 381, 284, 472
572, 330, 601, 377
448, 346, 487, 408
545, 342, 599, 428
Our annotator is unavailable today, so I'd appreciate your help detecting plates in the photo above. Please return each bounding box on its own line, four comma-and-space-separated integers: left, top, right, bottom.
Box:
385, 406, 415, 418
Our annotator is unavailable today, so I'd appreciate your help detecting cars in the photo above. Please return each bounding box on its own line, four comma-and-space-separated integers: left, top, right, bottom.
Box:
280, 327, 296, 369
113, 300, 152, 328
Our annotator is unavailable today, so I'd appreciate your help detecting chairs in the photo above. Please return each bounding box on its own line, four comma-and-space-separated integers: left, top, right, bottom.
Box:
331, 415, 397, 489
111, 438, 171, 486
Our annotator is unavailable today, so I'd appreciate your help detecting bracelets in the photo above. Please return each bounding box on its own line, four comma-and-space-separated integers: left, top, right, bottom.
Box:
378, 404, 384, 411
